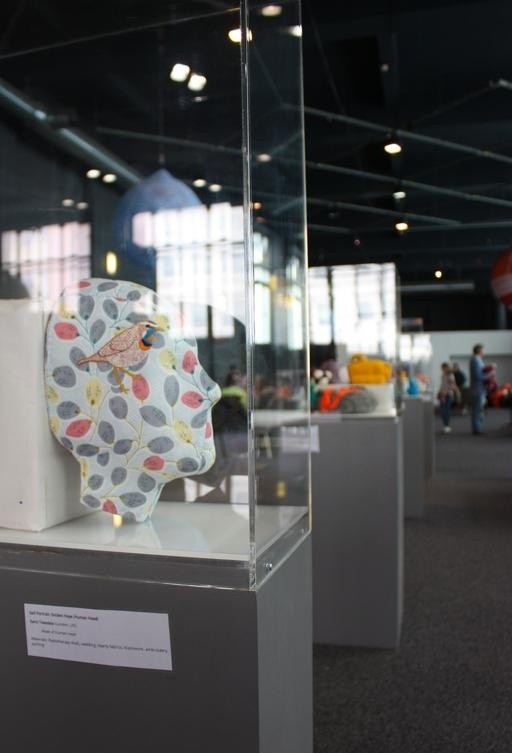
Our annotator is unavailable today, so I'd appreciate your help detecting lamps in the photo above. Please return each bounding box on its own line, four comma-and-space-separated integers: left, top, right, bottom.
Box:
378, 122, 414, 236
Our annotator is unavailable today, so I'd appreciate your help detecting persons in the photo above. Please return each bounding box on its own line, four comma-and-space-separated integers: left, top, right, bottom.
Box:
211, 370, 249, 496
436, 361, 457, 435
451, 361, 470, 416
467, 341, 496, 437
